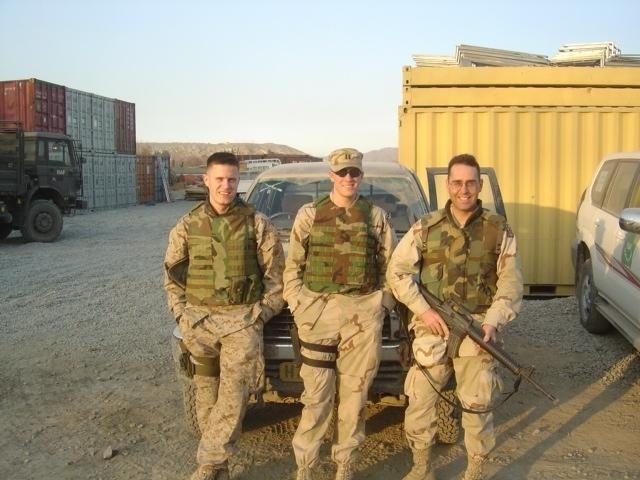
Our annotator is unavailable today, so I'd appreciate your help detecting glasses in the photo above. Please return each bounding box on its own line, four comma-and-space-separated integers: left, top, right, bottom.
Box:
333, 168, 361, 177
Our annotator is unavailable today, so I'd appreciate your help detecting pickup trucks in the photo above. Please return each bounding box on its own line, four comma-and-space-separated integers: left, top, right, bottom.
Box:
1, 117, 87, 239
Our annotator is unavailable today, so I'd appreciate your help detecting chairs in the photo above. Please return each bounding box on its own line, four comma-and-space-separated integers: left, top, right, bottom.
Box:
365, 193, 408, 219
278, 195, 312, 221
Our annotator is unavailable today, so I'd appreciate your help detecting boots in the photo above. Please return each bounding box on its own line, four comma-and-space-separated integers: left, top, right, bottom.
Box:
463, 454, 487, 480
401, 449, 436, 480
190, 463, 217, 480
297, 464, 316, 480
334, 462, 353, 480
217, 460, 230, 480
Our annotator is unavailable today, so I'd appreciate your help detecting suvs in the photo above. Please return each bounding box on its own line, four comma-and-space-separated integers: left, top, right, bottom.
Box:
574, 153, 640, 354
231, 163, 517, 403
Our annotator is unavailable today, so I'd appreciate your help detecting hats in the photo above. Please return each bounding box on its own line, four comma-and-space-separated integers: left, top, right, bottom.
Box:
328, 148, 363, 172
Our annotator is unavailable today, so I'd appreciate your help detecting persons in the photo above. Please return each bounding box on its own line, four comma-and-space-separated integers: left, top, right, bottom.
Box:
163, 152, 287, 480
283, 148, 399, 480
386, 154, 522, 480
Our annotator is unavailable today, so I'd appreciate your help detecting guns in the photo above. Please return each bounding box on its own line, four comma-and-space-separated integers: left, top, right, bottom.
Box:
394, 283, 560, 405
